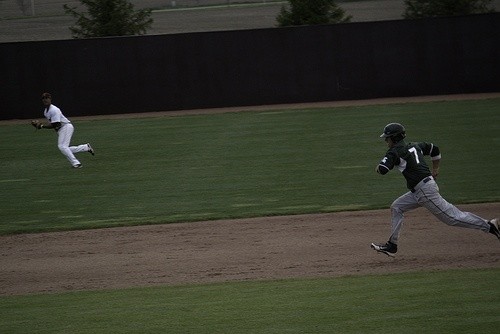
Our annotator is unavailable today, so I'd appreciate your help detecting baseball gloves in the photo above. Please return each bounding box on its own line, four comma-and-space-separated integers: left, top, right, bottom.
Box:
30, 119, 44, 130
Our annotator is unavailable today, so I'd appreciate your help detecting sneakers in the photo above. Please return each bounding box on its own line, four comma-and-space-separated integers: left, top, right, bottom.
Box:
488, 217, 500, 239
371, 241, 398, 257
87, 142, 94, 155
73, 164, 83, 168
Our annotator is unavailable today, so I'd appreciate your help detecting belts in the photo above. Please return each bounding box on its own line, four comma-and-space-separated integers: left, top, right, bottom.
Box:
411, 177, 430, 192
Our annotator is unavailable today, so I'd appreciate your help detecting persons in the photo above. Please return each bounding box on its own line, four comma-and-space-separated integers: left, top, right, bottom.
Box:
33, 93, 95, 168
371, 123, 500, 258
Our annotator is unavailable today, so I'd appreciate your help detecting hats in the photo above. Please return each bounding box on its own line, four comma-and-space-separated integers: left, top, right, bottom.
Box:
379, 122, 404, 138
